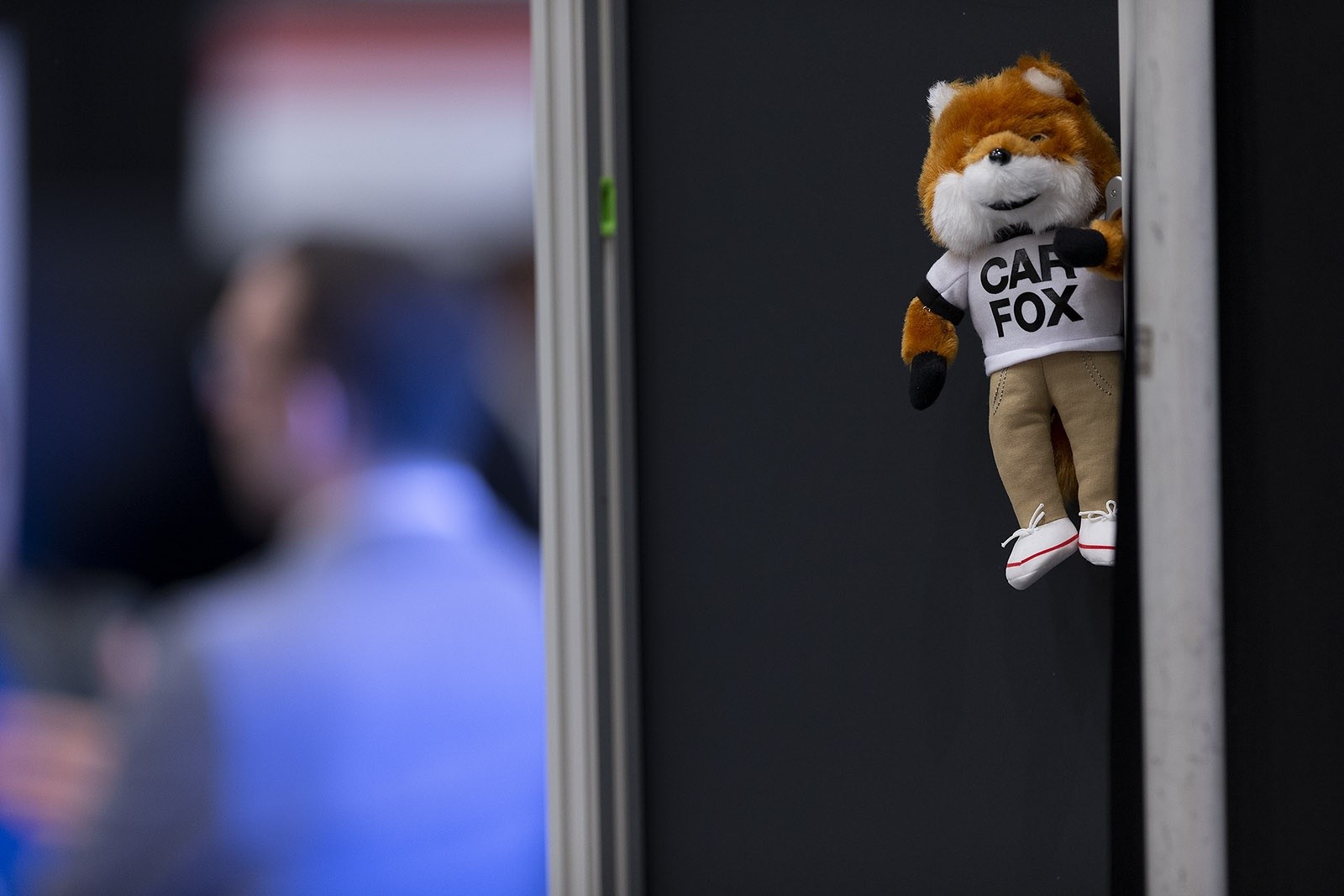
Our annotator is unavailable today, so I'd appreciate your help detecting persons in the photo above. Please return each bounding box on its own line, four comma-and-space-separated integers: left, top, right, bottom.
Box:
0, 240, 568, 896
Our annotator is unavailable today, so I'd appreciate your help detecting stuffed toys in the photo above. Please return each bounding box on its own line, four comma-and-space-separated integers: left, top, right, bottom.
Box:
897, 54, 1127, 590
